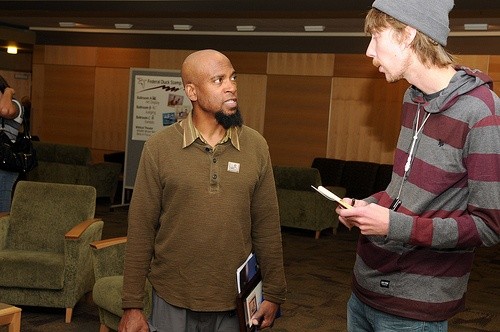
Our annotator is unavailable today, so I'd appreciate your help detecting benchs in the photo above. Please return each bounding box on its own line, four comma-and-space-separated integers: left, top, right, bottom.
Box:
310, 157, 393, 200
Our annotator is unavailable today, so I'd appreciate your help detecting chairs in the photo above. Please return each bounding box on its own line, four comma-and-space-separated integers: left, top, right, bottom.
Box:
270, 149, 346, 238
0, 142, 153, 332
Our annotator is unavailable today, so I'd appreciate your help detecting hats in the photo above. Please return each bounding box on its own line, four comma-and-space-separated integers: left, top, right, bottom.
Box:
371, 0, 455, 47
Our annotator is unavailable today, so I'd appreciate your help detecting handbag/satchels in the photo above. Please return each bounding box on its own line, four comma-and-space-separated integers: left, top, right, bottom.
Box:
0, 106, 38, 174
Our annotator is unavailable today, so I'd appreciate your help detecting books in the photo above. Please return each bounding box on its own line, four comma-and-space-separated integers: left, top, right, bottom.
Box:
236, 252, 264, 327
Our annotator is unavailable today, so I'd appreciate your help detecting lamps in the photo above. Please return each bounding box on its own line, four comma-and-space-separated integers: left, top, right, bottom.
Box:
235, 26, 256, 32
58, 22, 76, 27
305, 25, 326, 32
114, 23, 132, 28
465, 23, 488, 31
173, 24, 193, 30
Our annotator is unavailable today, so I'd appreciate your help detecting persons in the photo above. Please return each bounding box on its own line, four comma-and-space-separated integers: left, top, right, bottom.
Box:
0, 74, 23, 218
118, 49, 290, 332
336, 0, 500, 332
